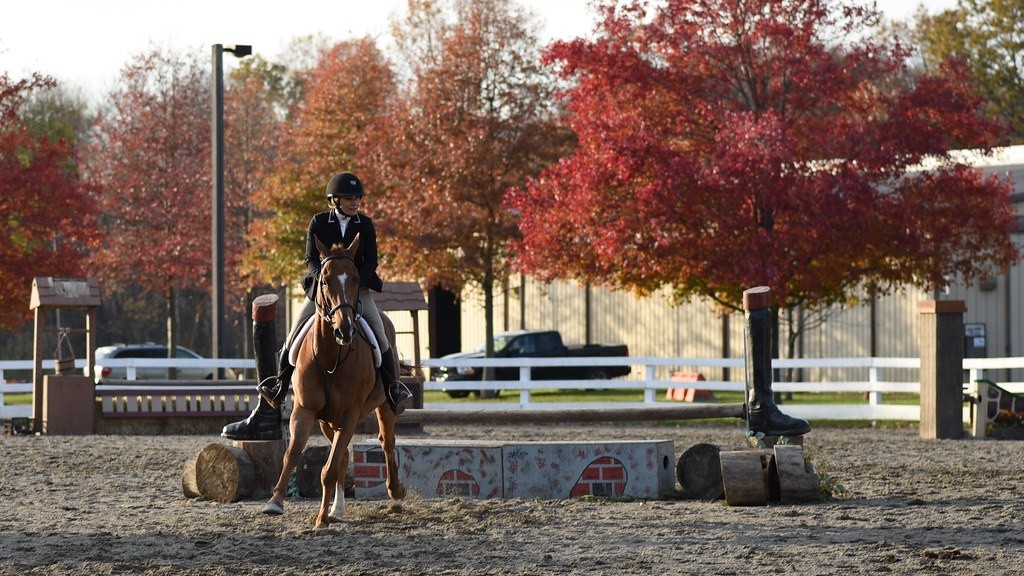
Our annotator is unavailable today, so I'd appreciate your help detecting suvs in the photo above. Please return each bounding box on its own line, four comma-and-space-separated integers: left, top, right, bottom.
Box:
83, 342, 238, 383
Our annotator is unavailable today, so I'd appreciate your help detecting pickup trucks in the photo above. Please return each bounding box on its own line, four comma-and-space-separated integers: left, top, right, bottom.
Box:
433, 331, 632, 398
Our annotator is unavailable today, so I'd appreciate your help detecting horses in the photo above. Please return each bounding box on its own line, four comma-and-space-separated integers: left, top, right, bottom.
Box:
260, 232, 408, 532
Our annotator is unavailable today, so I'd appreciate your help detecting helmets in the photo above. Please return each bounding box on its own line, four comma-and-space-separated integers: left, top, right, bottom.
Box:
326, 173, 363, 203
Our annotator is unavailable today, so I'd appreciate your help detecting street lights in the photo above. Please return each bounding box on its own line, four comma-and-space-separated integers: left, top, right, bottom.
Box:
209, 42, 254, 382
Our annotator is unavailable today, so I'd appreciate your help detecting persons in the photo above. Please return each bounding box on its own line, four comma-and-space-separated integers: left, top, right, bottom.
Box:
220, 293, 284, 443
262, 172, 411, 418
743, 286, 811, 438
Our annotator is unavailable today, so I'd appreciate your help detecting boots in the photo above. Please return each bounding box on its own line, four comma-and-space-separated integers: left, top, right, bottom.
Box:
744, 307, 811, 437
379, 346, 413, 416
220, 320, 282, 440
262, 342, 295, 404
